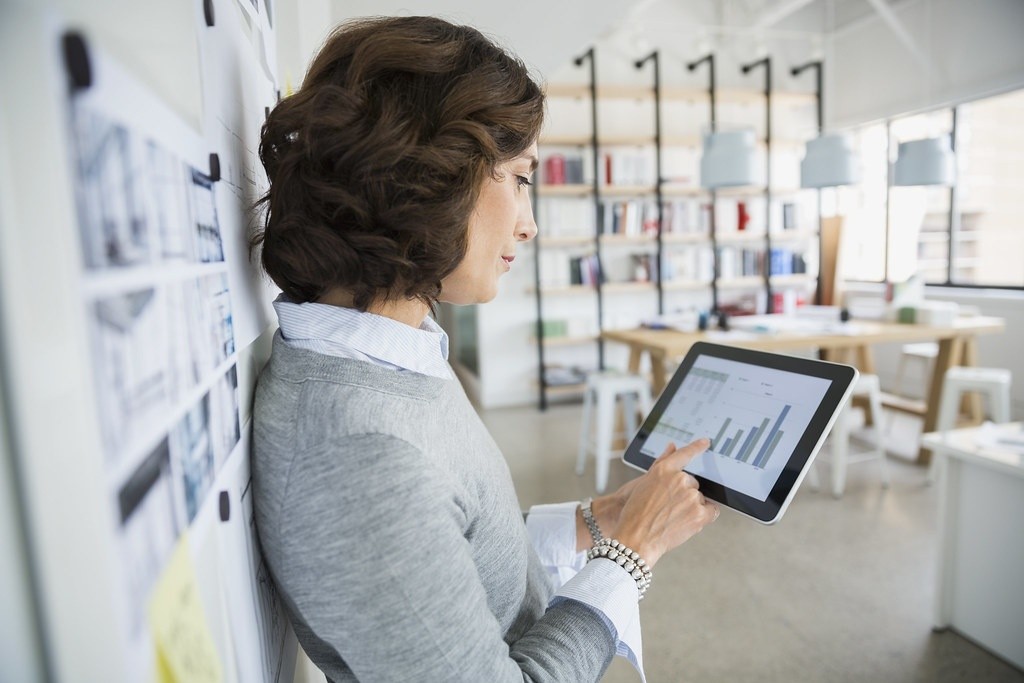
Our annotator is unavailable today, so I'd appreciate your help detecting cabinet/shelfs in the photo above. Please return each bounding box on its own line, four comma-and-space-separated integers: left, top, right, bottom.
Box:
525, 84, 840, 395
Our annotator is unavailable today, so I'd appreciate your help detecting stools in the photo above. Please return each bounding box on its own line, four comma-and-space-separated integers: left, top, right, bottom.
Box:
574, 368, 654, 494
925, 365, 1011, 478
893, 343, 939, 402
807, 372, 890, 500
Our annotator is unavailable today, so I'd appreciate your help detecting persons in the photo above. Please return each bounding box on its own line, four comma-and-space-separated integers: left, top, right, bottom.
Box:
246, 14, 718, 682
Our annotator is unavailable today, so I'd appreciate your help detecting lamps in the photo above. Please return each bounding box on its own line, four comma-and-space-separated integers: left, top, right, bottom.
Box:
700, 125, 767, 190
800, 0, 858, 188
892, 0, 956, 186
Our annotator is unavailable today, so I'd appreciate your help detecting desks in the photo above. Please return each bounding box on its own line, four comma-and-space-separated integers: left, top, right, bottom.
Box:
918, 420, 1024, 670
601, 314, 1005, 468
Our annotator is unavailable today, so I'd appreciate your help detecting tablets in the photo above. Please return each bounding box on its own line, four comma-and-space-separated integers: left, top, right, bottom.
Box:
620, 342, 860, 525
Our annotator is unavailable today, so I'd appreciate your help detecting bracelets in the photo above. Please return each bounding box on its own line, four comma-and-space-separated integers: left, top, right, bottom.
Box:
585, 537, 653, 602
580, 498, 603, 544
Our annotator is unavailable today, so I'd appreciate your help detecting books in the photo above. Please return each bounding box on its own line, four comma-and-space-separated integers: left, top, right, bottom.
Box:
539, 149, 815, 344
